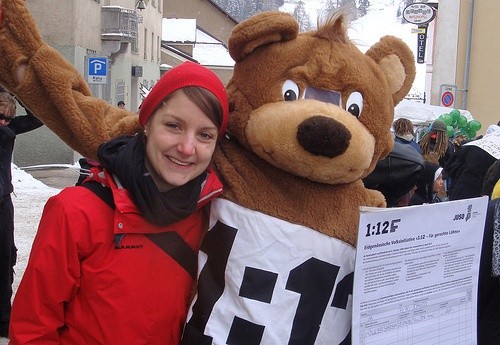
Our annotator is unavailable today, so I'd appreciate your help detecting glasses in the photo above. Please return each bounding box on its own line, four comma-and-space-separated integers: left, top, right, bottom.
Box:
0, 113, 13, 123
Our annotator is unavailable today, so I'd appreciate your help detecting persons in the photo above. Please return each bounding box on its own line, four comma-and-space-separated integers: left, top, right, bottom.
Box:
447, 130, 467, 152
0, 92, 17, 338
118, 101, 125, 110
476, 160, 500, 345
8, 63, 232, 344
364, 142, 425, 208
409, 162, 444, 206
414, 119, 454, 202
393, 118, 419, 151
445, 121, 500, 200
9, 94, 45, 135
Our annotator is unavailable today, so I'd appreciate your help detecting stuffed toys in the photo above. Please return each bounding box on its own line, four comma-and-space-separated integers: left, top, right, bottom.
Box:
1, 0, 416, 345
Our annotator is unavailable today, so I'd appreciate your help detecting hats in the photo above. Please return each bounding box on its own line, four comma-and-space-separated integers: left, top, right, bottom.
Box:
139, 62, 229, 136
431, 119, 447, 132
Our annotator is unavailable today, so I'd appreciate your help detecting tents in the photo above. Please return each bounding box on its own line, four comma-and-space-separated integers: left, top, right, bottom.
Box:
390, 98, 473, 138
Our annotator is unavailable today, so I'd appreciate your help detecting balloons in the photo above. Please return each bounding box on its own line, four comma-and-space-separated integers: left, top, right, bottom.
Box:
440, 110, 482, 140
